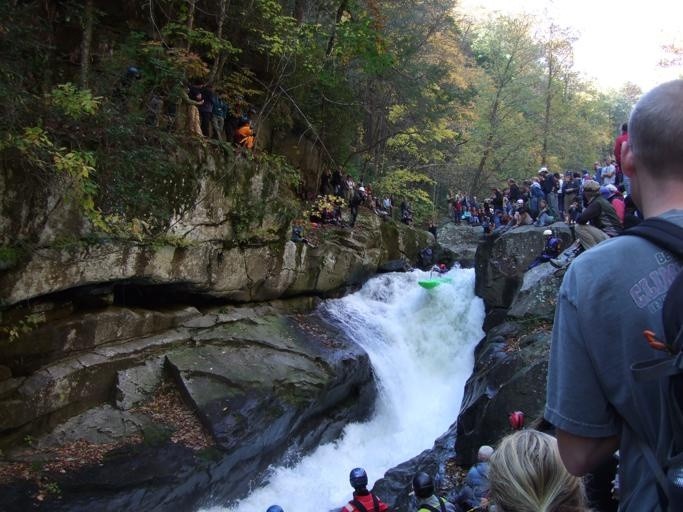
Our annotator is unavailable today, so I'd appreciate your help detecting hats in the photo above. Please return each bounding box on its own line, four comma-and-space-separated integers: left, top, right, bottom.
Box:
538, 167, 548, 172
583, 180, 602, 193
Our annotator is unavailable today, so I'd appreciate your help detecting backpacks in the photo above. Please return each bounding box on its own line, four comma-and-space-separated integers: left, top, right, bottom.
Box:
620, 216, 683, 512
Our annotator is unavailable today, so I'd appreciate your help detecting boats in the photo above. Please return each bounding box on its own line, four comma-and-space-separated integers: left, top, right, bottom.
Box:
419, 276, 454, 289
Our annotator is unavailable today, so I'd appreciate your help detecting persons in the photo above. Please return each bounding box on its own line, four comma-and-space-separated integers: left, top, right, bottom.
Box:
119, 64, 258, 150
428, 217, 437, 239
320, 164, 414, 227
417, 246, 451, 271
266, 467, 456, 512
447, 77, 683, 512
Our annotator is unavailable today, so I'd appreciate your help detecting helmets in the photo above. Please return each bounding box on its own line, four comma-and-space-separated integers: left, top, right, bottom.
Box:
543, 230, 553, 235
349, 467, 368, 488
510, 410, 526, 430
413, 471, 434, 497
477, 445, 495, 461
